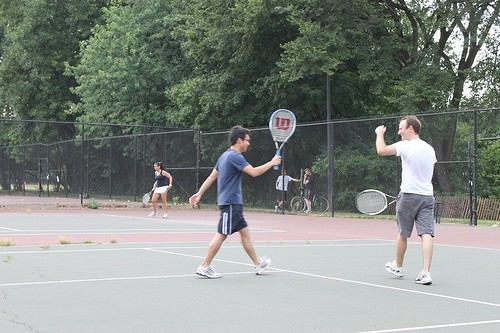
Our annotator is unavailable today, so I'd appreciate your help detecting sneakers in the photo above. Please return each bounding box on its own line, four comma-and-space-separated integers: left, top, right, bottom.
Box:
384, 260, 404, 278
196, 265, 222, 278
254, 257, 271, 275
414, 272, 433, 285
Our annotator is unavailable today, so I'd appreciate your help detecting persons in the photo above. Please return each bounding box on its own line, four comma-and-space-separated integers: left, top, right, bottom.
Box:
375, 115, 438, 284
149, 161, 172, 218
189, 126, 282, 279
303, 168, 314, 213
275, 169, 300, 212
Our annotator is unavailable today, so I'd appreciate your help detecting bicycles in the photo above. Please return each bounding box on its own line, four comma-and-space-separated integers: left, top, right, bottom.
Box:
290, 187, 329, 213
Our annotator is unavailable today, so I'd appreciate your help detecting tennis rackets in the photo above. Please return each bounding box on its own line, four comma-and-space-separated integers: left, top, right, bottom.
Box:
142, 187, 155, 205
354, 189, 396, 217
267, 108, 297, 171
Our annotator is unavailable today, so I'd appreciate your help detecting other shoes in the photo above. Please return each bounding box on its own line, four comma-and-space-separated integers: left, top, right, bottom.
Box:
162, 213, 168, 218
149, 212, 157, 217
303, 210, 312, 213
275, 206, 278, 214
